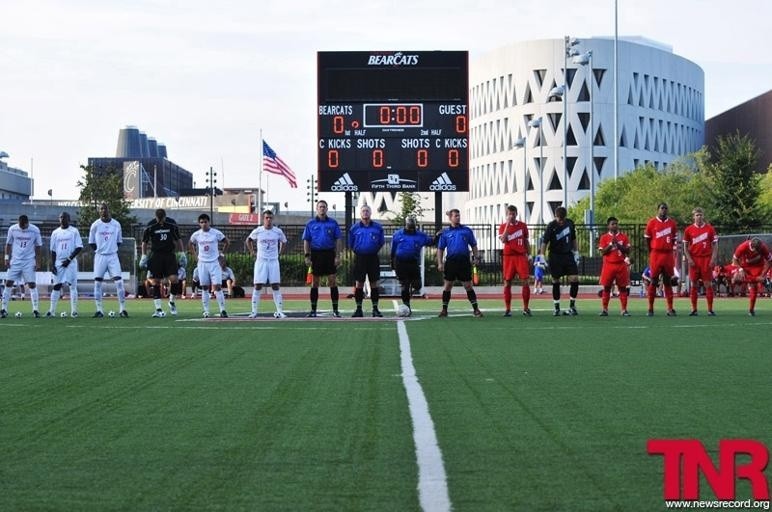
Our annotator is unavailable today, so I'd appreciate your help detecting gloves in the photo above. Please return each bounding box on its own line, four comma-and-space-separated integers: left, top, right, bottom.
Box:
573, 253, 582, 266
176, 251, 187, 268
138, 255, 149, 269
538, 257, 549, 271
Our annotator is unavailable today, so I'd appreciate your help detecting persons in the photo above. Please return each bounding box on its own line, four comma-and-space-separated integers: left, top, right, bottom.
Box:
87, 203, 130, 317
12, 279, 26, 301
347, 204, 385, 317
437, 209, 484, 318
538, 206, 581, 316
46, 211, 84, 318
302, 200, 342, 317
139, 208, 237, 317
390, 215, 442, 316
1, 213, 43, 318
597, 203, 772, 316
499, 205, 534, 316
246, 209, 288, 318
532, 250, 544, 294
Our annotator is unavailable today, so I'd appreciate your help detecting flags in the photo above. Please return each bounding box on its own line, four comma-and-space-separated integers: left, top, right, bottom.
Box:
262, 139, 297, 188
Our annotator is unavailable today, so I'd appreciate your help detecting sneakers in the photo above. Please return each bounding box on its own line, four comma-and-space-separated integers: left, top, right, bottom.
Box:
0, 308, 762, 323
533, 289, 545, 295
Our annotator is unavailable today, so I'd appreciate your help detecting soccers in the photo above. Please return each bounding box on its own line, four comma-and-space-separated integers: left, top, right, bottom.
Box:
16, 312, 22, 318
108, 311, 114, 317
160, 311, 165, 318
395, 305, 409, 317
273, 311, 279, 317
203, 311, 209, 318
61, 312, 67, 318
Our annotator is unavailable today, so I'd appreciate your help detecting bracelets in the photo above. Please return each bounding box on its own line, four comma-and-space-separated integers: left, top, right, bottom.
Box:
305, 252, 310, 257
4, 254, 10, 260
473, 253, 479, 259
68, 257, 72, 262
335, 250, 341, 257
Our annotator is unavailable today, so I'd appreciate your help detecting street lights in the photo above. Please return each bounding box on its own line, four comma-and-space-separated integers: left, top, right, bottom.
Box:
284, 201, 289, 215
574, 50, 596, 258
528, 115, 544, 224
552, 34, 583, 213
332, 203, 337, 217
231, 198, 237, 212
205, 166, 217, 227
306, 174, 319, 219
175, 194, 179, 207
351, 195, 358, 225
514, 136, 528, 225
47, 189, 53, 206
